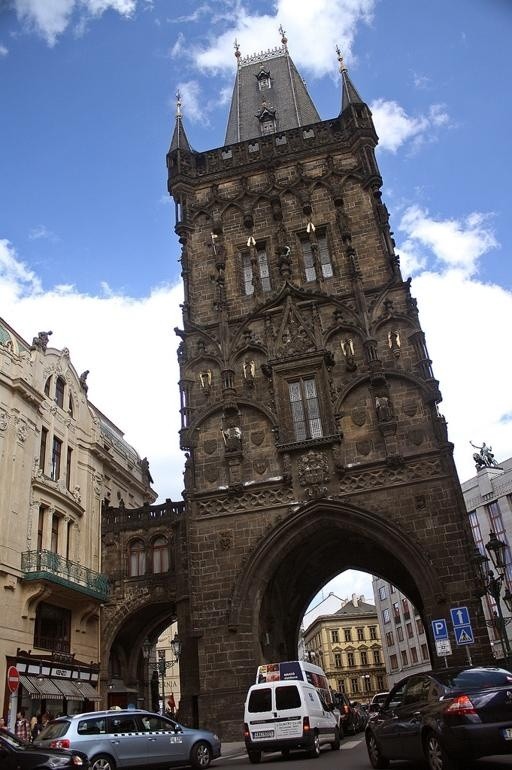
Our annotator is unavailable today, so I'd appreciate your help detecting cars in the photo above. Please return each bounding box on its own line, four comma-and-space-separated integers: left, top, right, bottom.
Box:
0, 709, 221, 770
334, 665, 512, 770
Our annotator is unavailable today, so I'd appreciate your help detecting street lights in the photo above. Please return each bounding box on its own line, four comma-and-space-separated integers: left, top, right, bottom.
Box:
144, 632, 183, 713
470, 528, 512, 668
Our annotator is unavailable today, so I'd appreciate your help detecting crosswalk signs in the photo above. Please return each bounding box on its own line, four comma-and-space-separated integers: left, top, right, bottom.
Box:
454, 626, 473, 645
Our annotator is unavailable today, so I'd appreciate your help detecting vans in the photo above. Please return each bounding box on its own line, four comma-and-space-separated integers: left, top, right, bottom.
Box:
243, 660, 340, 763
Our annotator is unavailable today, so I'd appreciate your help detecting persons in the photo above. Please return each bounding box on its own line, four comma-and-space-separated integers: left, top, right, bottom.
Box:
39, 331, 52, 351
80, 370, 89, 394
469, 441, 499, 466
183, 453, 192, 489
0, 711, 55, 742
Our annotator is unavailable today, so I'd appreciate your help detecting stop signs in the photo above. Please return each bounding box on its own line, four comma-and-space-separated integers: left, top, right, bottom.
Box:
7, 666, 21, 693
